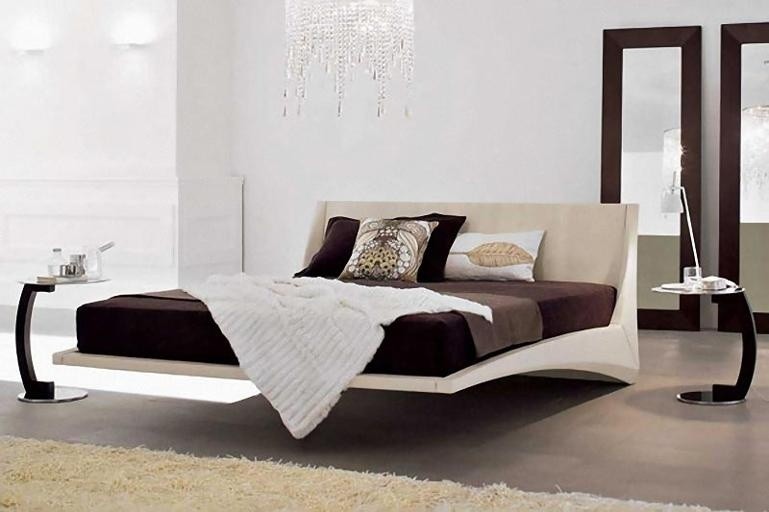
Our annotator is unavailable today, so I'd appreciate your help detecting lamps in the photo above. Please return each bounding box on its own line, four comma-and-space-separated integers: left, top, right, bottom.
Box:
282, 0, 415, 115
661, 185, 701, 288
741, 106, 769, 189
664, 128, 680, 172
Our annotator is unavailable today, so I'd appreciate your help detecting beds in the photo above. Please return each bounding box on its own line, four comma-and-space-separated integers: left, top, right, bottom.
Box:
54, 201, 640, 396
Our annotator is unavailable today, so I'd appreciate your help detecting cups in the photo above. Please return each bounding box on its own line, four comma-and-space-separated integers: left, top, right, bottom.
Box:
683, 266, 703, 289
60, 265, 78, 278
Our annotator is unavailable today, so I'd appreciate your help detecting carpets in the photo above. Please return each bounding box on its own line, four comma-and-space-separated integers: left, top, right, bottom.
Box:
0, 436, 719, 512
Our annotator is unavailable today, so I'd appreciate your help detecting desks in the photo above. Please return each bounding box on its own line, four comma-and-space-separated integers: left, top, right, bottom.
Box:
654, 285, 758, 405
16, 276, 113, 405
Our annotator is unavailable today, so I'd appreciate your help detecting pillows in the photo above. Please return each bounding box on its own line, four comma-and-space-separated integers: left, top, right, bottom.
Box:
338, 216, 438, 283
446, 228, 545, 282
292, 212, 464, 283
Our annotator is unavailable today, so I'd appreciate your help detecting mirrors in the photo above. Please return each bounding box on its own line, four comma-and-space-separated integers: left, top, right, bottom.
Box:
718, 22, 769, 335
601, 25, 702, 332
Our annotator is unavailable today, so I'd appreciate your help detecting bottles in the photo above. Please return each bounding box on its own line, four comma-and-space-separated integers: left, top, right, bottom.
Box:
48, 248, 67, 276
69, 253, 86, 277
